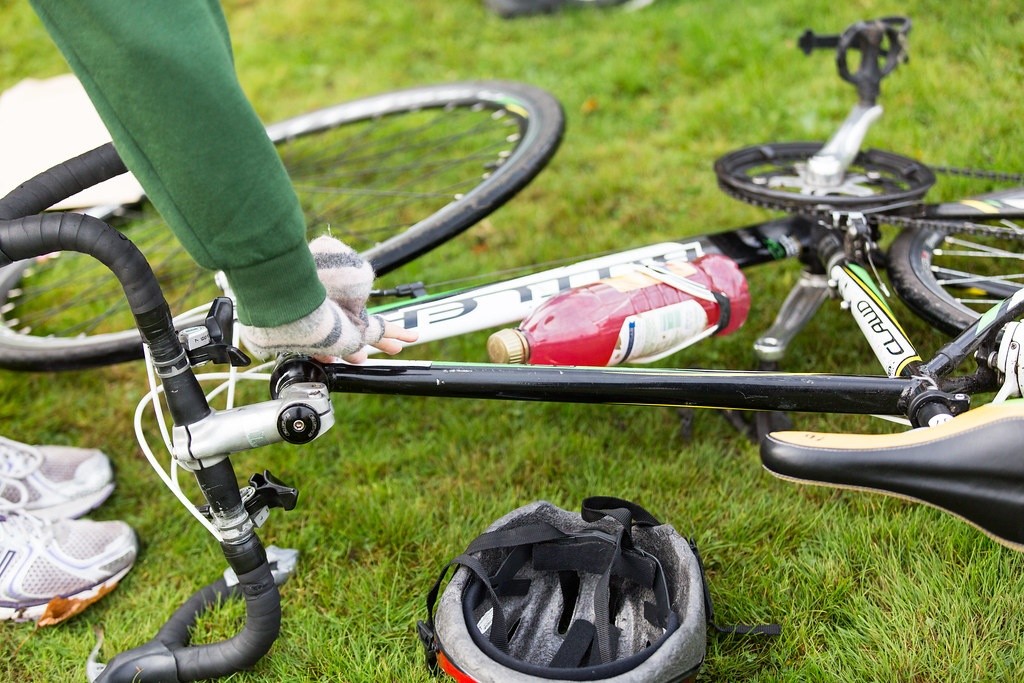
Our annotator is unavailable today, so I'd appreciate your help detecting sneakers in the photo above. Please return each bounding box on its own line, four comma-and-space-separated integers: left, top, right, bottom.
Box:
2, 434, 138, 627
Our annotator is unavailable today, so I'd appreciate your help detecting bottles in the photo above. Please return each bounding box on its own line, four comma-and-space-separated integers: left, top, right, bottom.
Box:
489, 251, 750, 369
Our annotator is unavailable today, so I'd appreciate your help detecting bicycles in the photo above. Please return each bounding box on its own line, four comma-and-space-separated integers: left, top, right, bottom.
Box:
0, 13, 1023, 683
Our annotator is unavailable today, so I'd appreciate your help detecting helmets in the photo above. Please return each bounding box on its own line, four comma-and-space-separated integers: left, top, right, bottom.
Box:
416, 495, 777, 683
